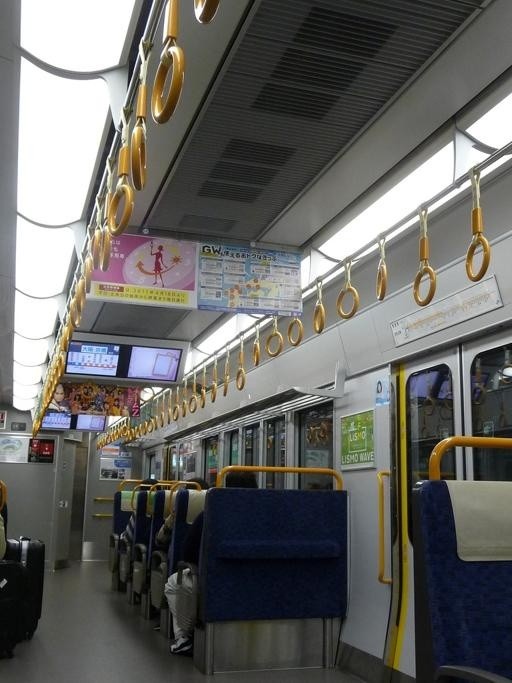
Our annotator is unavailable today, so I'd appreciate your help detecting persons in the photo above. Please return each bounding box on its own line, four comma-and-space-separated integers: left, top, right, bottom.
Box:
121, 477, 159, 545
48, 382, 124, 416
151, 243, 168, 288
0, 514, 6, 560
165, 463, 259, 655
152, 477, 208, 631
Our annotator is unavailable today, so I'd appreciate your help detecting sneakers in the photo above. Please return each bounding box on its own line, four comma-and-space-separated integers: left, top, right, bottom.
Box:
169, 636, 192, 653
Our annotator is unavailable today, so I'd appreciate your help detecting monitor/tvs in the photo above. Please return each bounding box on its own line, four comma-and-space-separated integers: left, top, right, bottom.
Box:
40, 411, 107, 432
63, 332, 190, 388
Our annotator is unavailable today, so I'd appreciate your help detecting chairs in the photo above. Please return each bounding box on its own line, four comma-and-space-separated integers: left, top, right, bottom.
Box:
411, 436, 511, 683
111, 478, 207, 642
175, 464, 349, 676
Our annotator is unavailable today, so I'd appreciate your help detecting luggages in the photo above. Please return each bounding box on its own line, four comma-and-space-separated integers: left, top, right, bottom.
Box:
0, 562, 42, 657
5, 532, 46, 639
